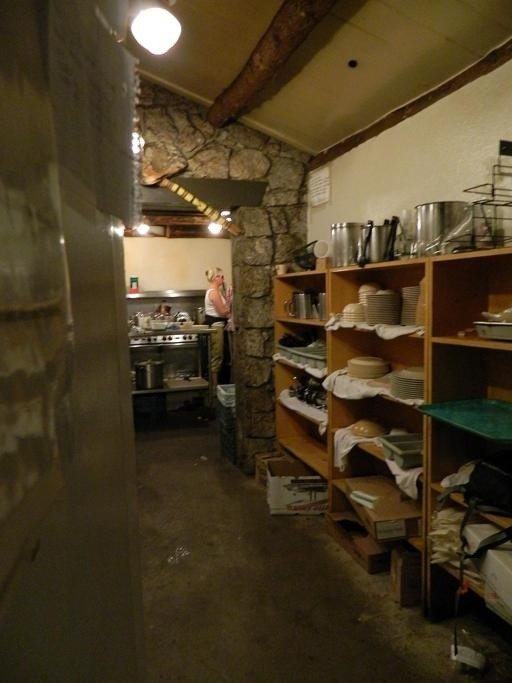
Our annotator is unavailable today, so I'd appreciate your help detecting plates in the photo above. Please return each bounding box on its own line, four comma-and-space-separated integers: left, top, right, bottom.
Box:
390, 364, 427, 400
366, 283, 431, 327
347, 354, 393, 382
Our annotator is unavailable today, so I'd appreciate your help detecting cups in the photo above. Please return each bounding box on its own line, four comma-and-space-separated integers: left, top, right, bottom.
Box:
288, 292, 326, 321
276, 263, 289, 276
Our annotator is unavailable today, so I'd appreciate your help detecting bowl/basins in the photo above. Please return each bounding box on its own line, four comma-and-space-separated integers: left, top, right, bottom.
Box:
340, 280, 380, 323
351, 415, 393, 439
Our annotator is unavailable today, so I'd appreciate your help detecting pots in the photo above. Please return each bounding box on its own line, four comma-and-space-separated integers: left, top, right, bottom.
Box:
330, 220, 396, 268
196, 306, 207, 324
413, 200, 473, 256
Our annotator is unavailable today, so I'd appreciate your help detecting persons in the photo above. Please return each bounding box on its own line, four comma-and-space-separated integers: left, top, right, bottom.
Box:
202, 266, 234, 398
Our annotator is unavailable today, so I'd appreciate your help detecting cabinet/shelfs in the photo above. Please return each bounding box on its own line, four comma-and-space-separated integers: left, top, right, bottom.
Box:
329, 258, 428, 604
428, 248, 512, 626
274, 258, 329, 511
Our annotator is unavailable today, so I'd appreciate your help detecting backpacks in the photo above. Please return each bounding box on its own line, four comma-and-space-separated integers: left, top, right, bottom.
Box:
463, 461, 512, 518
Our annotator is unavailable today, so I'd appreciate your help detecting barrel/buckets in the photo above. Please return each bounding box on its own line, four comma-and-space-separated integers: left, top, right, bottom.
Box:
414, 200, 471, 248
329, 220, 366, 267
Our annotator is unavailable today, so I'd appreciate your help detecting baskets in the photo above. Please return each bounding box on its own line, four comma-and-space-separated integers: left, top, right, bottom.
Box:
138, 317, 194, 330
217, 384, 236, 466
